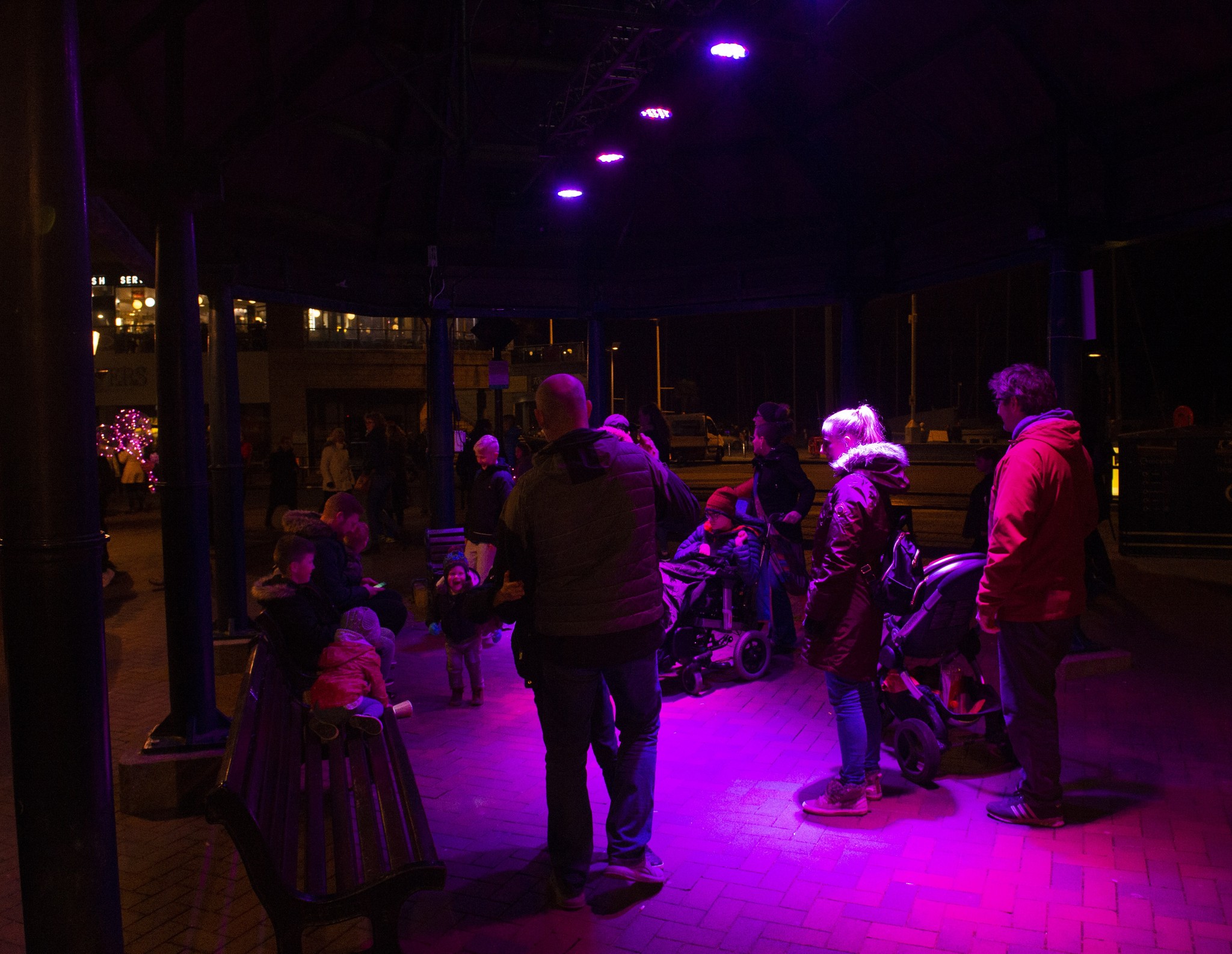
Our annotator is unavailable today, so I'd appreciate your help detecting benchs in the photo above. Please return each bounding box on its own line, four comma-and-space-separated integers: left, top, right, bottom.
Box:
203, 643, 447, 954
423, 528, 467, 579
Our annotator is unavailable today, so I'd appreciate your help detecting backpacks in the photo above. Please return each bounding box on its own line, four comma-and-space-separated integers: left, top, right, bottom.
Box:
856, 490, 926, 615
98, 455, 112, 482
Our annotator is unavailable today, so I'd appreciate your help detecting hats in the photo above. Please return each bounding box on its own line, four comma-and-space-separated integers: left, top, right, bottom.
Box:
442, 545, 469, 574
758, 402, 787, 422
340, 606, 381, 644
705, 486, 738, 519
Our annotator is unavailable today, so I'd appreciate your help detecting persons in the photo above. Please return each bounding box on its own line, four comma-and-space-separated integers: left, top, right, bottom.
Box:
117, 439, 145, 511
454, 413, 533, 494
660, 486, 762, 677
425, 551, 490, 705
966, 447, 998, 554
480, 375, 702, 883
463, 435, 515, 623
264, 415, 418, 545
802, 405, 912, 816
119, 323, 154, 352
264, 496, 408, 740
732, 425, 754, 452
973, 366, 1100, 828
733, 402, 815, 655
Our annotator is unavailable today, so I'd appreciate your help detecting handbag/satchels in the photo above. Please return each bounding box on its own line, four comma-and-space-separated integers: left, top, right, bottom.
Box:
766, 532, 810, 596
1085, 529, 1115, 594
354, 472, 372, 492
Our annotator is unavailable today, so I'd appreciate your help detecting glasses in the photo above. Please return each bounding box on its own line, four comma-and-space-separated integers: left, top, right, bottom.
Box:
990, 397, 1006, 404
755, 413, 763, 418
706, 511, 720, 518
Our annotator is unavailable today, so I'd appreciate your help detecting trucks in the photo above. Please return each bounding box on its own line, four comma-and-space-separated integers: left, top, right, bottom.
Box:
664, 414, 724, 466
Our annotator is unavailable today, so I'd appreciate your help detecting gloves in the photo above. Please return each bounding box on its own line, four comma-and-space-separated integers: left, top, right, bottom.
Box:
804, 616, 825, 635
492, 629, 501, 643
327, 482, 333, 487
428, 622, 439, 635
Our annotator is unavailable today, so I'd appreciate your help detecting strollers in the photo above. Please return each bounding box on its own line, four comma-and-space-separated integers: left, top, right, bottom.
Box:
877, 532, 1017, 784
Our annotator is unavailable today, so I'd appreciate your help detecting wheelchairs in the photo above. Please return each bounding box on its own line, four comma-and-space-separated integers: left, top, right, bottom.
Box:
657, 499, 787, 695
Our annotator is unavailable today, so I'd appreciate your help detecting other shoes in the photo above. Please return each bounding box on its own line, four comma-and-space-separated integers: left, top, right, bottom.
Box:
350, 713, 383, 735
378, 535, 385, 539
551, 871, 586, 907
471, 691, 480, 705
386, 537, 395, 543
602, 854, 663, 883
308, 717, 338, 740
450, 693, 462, 706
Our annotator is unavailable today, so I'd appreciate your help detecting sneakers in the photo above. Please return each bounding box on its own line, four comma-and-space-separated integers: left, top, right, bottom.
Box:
803, 779, 868, 816
866, 771, 882, 800
986, 790, 1064, 827
